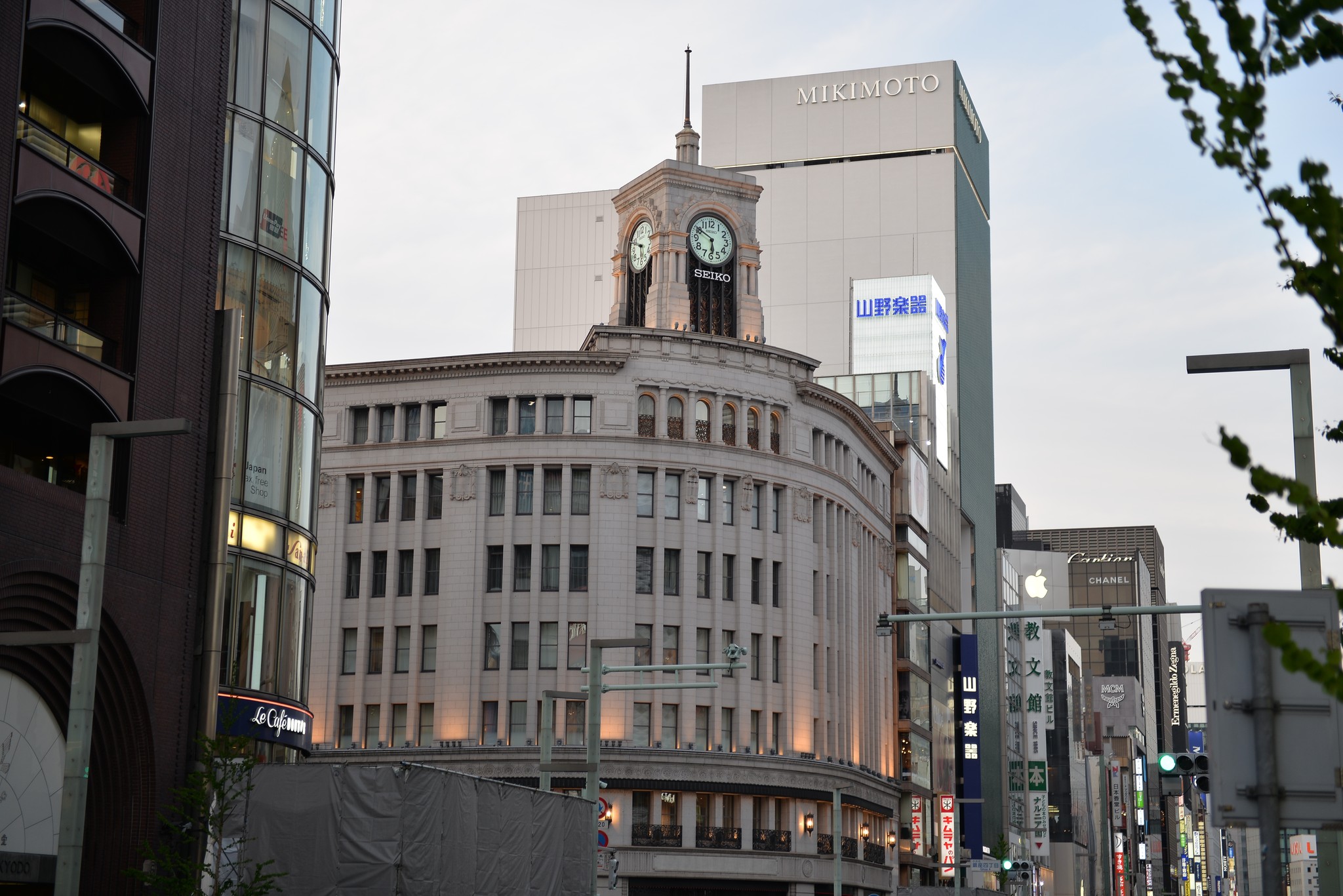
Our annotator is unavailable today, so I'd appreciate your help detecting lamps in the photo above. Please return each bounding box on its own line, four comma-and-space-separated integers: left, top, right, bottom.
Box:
860, 820, 869, 844
606, 799, 613, 829
887, 830, 896, 852
804, 809, 814, 838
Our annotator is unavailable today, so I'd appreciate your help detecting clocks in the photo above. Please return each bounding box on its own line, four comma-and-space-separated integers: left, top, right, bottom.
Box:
688, 212, 736, 267
627, 218, 654, 273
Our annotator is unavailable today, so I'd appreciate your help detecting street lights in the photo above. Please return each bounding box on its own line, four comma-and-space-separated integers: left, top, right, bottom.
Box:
954, 798, 985, 896
833, 782, 855, 896
1075, 852, 1098, 896
1161, 892, 1176, 896
1184, 349, 1343, 896
1117, 873, 1136, 896
52, 417, 194, 896
1021, 828, 1047, 896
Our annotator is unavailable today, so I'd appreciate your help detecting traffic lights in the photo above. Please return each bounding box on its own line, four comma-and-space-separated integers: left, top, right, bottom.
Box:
1002, 860, 1034, 871
1020, 871, 1030, 881
1196, 774, 1209, 794
608, 858, 619, 890
1158, 752, 1209, 777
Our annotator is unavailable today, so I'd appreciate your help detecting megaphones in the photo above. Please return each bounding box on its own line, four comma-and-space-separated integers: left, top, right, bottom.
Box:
740, 647, 747, 655
